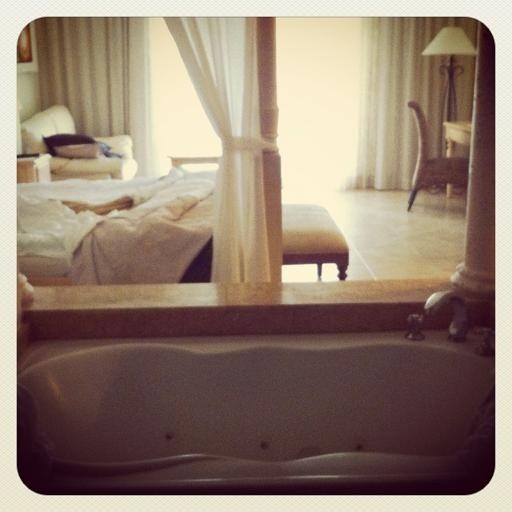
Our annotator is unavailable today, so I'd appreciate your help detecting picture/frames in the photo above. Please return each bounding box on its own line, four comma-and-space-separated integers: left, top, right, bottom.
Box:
16, 21, 39, 73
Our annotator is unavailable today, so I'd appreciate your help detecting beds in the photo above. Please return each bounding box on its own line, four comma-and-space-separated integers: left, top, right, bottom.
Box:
17, 175, 213, 285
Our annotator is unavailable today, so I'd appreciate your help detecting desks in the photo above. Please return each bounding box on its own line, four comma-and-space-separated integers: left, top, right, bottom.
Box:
442, 119, 472, 198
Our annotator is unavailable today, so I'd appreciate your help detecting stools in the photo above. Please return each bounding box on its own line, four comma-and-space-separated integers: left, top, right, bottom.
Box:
280, 203, 350, 281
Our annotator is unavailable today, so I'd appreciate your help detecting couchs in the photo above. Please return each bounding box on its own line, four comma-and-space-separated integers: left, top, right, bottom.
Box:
19, 104, 138, 180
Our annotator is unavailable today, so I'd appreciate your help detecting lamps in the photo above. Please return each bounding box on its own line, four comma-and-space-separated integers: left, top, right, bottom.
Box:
421, 27, 479, 193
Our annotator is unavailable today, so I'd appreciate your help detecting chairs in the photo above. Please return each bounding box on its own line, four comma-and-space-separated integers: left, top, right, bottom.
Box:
407, 101, 469, 211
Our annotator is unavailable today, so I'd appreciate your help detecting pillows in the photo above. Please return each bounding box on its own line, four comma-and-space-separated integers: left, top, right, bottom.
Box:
42, 133, 111, 159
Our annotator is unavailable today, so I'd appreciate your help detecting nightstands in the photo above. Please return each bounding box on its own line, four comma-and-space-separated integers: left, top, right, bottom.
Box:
17, 153, 52, 183
